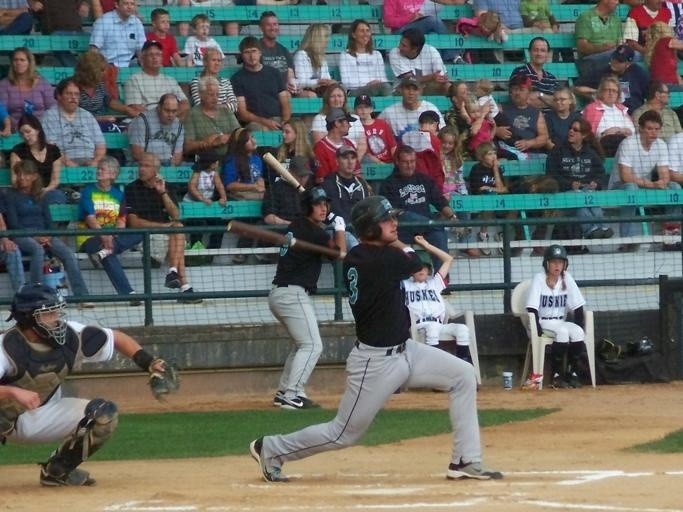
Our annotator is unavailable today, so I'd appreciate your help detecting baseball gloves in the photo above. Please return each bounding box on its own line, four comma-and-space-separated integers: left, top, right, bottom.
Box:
147, 357, 183, 406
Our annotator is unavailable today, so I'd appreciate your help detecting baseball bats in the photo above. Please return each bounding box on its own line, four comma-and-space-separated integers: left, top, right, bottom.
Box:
263, 152, 306, 193
226, 220, 348, 259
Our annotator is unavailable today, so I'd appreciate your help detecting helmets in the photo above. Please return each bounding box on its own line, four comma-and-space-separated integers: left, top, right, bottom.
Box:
626, 335, 653, 356
412, 250, 433, 274
542, 244, 569, 273
349, 195, 405, 240
595, 334, 625, 366
301, 184, 334, 205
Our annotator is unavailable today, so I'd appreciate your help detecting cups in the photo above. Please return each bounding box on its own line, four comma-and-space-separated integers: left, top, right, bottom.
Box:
503, 371, 513, 391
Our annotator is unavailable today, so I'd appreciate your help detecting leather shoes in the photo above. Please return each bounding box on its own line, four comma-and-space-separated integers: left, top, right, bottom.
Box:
440, 288, 451, 295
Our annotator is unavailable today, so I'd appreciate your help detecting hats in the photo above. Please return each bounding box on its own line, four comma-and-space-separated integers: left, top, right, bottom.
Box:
336, 144, 357, 157
418, 110, 440, 125
325, 106, 358, 126
391, 77, 418, 91
508, 70, 532, 88
354, 94, 372, 109
239, 36, 262, 52
608, 44, 636, 64
191, 149, 221, 172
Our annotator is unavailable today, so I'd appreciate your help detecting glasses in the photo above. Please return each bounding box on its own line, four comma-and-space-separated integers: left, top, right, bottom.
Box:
569, 125, 583, 133
142, 50, 162, 57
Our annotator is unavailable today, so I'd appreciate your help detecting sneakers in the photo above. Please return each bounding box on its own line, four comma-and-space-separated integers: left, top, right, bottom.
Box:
278, 395, 321, 411
445, 458, 506, 482
254, 253, 277, 264
269, 387, 284, 403
568, 373, 580, 390
129, 298, 142, 306
459, 248, 479, 258
548, 376, 568, 388
232, 241, 252, 264
495, 230, 508, 255
164, 270, 182, 288
38, 455, 97, 488
476, 231, 491, 259
141, 254, 162, 269
248, 431, 291, 484
175, 286, 204, 305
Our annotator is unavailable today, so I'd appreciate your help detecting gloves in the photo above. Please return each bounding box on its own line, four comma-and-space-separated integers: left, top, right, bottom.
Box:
331, 215, 346, 232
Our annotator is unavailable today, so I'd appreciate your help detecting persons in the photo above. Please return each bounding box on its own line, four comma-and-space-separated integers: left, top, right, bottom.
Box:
579, 80, 635, 156
389, 28, 454, 96
645, 23, 683, 89
352, 92, 396, 162
77, 158, 144, 309
445, 83, 492, 164
0, 286, 177, 485
324, 147, 372, 255
191, 49, 238, 114
67, 53, 143, 131
467, 80, 528, 151
526, 243, 585, 389
2, 1, 33, 37
521, 0, 569, 62
90, 1, 148, 70
292, 24, 342, 94
0, 101, 10, 174
511, 39, 573, 109
666, 132, 683, 237
625, 1, 671, 55
167, 0, 240, 36
231, 37, 291, 135
269, 188, 346, 411
579, 46, 651, 115
185, 78, 242, 164
422, 127, 473, 253
311, 86, 366, 165
75, 46, 118, 119
10, 115, 68, 241
248, 196, 504, 482
461, 101, 494, 157
609, 113, 679, 247
0, 214, 27, 303
207, 125, 264, 265
384, 0, 465, 64
476, 1, 532, 60
379, 77, 447, 149
415, 110, 439, 139
263, 158, 321, 256
94, 0, 120, 21
34, 79, 109, 169
545, 121, 613, 237
183, 153, 228, 263
394, 235, 470, 367
184, 15, 224, 65
128, 95, 186, 200
376, 147, 455, 291
631, 82, 679, 143
136, 8, 183, 68
4, 48, 56, 131
575, 0, 649, 84
340, 19, 394, 95
542, 89, 587, 151
484, 82, 549, 156
127, 41, 188, 123
37, 2, 90, 34
270, 119, 313, 179
469, 140, 515, 255
254, 11, 316, 98
126, 154, 205, 305
2, 160, 92, 307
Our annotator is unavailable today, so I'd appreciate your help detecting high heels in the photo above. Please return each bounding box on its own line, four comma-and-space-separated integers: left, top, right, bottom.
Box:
77, 301, 96, 309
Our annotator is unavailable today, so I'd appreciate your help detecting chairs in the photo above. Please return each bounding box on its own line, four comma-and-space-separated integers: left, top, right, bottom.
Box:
510, 279, 597, 392
405, 299, 483, 391
